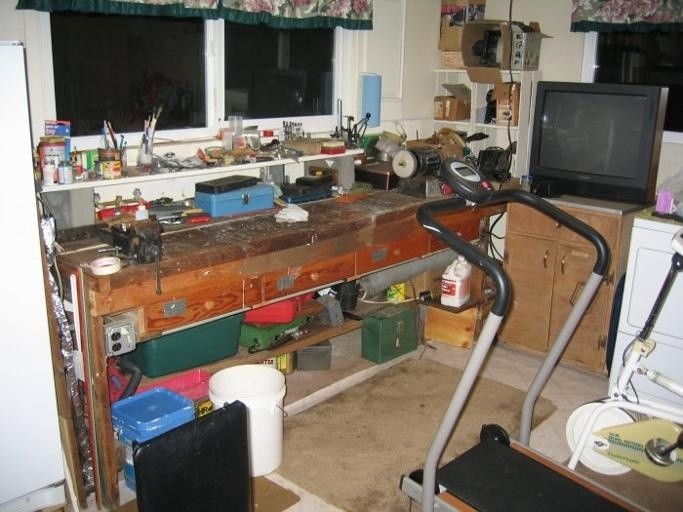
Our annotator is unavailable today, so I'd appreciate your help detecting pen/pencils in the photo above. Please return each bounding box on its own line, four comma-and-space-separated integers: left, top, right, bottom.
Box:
101, 120, 127, 151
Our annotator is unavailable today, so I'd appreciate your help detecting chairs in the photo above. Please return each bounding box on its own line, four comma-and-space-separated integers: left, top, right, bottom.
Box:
130, 400, 252, 511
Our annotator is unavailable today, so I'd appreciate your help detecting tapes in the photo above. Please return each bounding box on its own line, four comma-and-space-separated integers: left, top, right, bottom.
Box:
90, 256, 121, 276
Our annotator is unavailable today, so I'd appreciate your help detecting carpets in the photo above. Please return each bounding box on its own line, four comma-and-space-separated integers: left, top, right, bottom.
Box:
109, 472, 301, 510
274, 348, 557, 512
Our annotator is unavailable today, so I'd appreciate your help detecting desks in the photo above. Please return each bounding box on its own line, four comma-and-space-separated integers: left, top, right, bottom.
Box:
45, 174, 521, 511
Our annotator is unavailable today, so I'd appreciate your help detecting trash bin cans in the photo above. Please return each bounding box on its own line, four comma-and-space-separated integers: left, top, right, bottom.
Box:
112, 386, 196, 493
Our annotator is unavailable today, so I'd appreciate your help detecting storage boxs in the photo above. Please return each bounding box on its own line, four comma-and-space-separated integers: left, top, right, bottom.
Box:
361, 299, 417, 365
405, 139, 465, 177
438, 0, 553, 81
193, 184, 274, 217
423, 298, 496, 350
405, 268, 445, 299
433, 83, 471, 122
119, 311, 244, 378
296, 338, 332, 370
490, 81, 521, 127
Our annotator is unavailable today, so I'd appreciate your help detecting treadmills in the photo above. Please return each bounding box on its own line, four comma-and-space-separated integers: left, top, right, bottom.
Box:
400, 158, 651, 511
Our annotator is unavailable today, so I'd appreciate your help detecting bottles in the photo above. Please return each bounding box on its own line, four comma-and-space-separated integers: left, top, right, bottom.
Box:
33, 136, 122, 183
265, 351, 295, 375
362, 73, 382, 126
135, 199, 150, 220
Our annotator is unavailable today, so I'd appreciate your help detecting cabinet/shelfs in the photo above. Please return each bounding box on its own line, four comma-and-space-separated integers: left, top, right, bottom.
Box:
39, 147, 365, 229
607, 217, 683, 409
432, 68, 543, 184
496, 199, 635, 371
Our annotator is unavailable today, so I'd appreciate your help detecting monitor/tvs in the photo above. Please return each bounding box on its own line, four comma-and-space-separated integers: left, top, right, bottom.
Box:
527, 80, 669, 205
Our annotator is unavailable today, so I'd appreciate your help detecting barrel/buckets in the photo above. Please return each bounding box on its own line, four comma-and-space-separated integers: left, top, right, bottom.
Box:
208, 365, 288, 479
440, 254, 473, 308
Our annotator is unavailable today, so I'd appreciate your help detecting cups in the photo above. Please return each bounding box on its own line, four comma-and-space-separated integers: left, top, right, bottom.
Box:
228, 114, 243, 139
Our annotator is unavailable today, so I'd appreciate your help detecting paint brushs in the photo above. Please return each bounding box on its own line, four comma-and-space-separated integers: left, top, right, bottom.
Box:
141, 106, 163, 154
